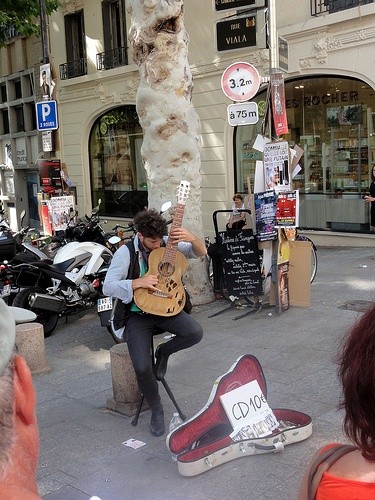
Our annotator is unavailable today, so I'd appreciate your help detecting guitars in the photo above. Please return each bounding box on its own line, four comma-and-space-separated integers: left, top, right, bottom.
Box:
133, 180, 191, 317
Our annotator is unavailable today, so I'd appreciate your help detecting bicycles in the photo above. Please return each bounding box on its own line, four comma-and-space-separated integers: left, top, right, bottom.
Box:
234, 235, 319, 305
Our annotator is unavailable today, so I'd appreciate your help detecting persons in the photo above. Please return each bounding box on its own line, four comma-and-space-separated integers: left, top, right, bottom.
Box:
364, 165, 375, 260
40, 71, 50, 98
102, 209, 207, 436
226, 195, 246, 231
299, 306, 375, 500
0, 298, 45, 500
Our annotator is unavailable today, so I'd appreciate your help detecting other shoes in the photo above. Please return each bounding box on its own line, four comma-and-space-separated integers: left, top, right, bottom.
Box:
150, 402, 165, 436
155, 342, 169, 381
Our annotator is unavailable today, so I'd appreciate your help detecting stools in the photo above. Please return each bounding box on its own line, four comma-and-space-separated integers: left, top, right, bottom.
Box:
131, 328, 187, 426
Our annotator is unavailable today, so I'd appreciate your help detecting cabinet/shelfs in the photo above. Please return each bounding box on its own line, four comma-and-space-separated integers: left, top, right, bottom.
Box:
304, 143, 329, 192
323, 142, 371, 192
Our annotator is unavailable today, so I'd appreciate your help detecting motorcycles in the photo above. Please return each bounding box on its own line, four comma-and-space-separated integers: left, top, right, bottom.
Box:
0, 199, 174, 339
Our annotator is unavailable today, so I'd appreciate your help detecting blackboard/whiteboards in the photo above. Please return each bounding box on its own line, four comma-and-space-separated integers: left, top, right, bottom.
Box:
215, 228, 262, 297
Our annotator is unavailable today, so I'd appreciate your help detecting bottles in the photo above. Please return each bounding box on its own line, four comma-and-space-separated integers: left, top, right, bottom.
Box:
169, 413, 184, 462
